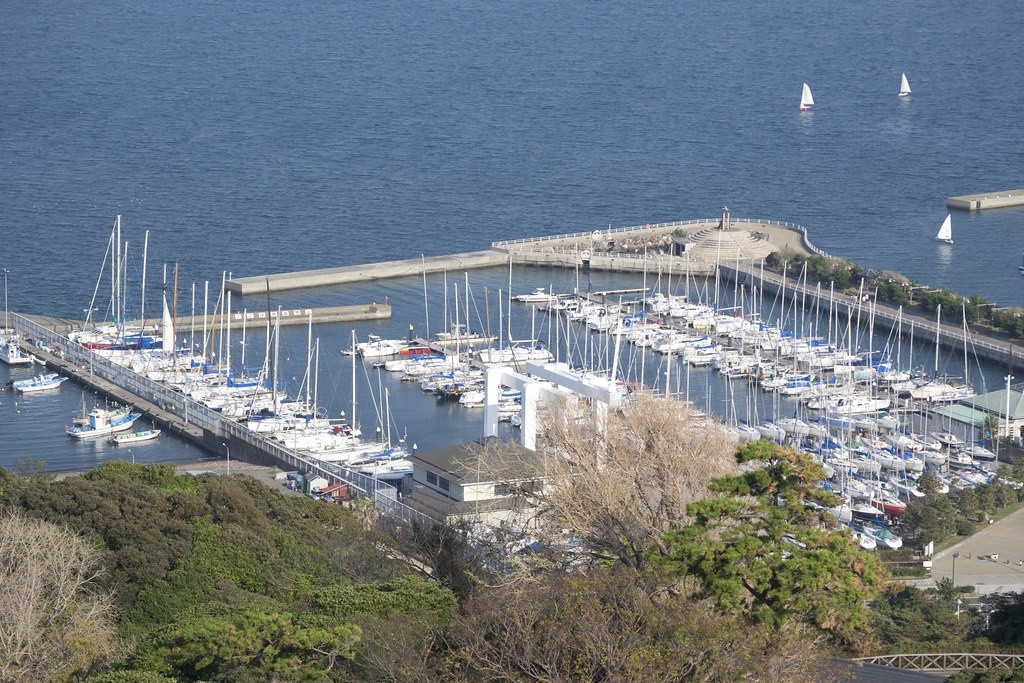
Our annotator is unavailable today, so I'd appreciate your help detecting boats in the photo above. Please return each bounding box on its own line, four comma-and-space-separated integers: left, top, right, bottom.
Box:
11, 372, 69, 393
67, 214, 415, 480
339, 227, 997, 550
64, 391, 142, 438
0, 329, 33, 364
63, 390, 135, 424
106, 428, 162, 444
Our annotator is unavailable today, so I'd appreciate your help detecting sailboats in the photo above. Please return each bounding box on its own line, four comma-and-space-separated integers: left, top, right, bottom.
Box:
897, 72, 912, 97
799, 83, 815, 111
934, 212, 954, 244
